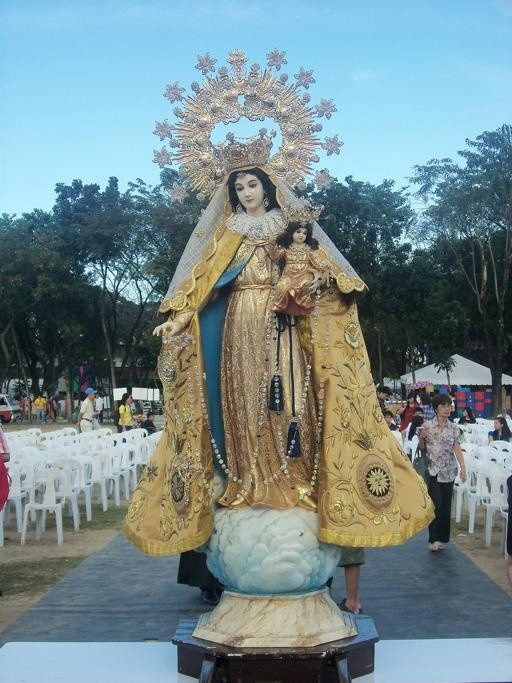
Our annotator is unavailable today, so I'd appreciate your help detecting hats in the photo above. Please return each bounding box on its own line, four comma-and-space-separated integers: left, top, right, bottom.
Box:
85, 387, 98, 395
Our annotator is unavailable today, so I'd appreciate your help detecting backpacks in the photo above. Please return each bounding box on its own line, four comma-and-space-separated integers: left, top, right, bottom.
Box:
113, 403, 126, 426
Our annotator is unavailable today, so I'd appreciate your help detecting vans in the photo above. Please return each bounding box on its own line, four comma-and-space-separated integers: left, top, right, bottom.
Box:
0, 394, 21, 423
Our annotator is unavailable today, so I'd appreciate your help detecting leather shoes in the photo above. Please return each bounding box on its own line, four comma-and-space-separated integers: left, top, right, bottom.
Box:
201, 588, 219, 604
81, 418, 92, 422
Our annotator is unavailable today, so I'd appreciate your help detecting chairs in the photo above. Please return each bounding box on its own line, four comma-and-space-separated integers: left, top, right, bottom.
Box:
390, 415, 512, 556
0, 424, 164, 551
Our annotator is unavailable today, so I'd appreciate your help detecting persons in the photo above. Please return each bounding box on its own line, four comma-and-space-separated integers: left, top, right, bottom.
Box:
14, 387, 161, 438
268, 220, 328, 316
504, 476, 512, 587
0, 421, 10, 594
373, 385, 512, 552
150, 167, 330, 513
325, 544, 364, 616
175, 546, 227, 605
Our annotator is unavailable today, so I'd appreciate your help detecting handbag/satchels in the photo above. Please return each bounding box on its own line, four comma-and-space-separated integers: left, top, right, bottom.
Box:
413, 458, 430, 489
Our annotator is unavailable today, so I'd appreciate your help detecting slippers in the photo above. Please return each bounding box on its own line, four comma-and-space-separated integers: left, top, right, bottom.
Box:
337, 598, 362, 614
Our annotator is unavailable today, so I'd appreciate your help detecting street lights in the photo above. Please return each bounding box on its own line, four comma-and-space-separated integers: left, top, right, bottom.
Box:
60, 333, 86, 423
